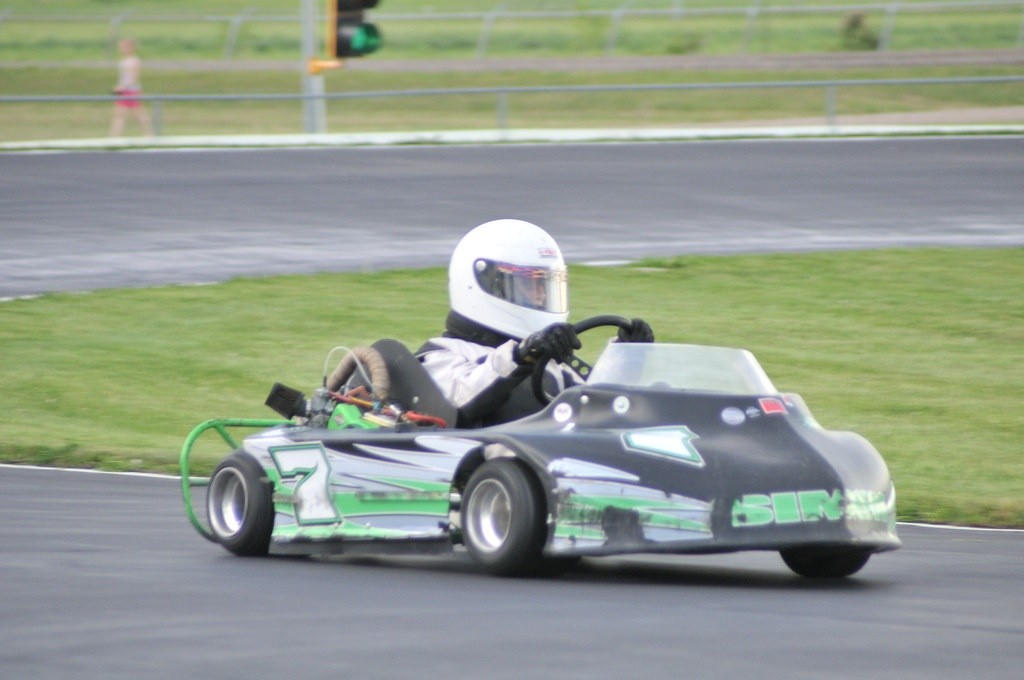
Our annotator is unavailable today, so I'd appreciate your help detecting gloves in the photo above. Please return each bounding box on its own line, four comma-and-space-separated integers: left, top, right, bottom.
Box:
513, 322, 582, 366
617, 318, 654, 343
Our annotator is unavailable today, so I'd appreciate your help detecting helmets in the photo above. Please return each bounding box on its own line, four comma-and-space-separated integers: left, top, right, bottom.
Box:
447, 218, 569, 342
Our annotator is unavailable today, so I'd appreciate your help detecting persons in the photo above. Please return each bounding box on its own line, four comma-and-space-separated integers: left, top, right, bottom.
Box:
413, 218, 655, 429
108, 37, 157, 138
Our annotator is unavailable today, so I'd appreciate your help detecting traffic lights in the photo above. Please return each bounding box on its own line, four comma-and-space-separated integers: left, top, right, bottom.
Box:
336, 0, 384, 59
